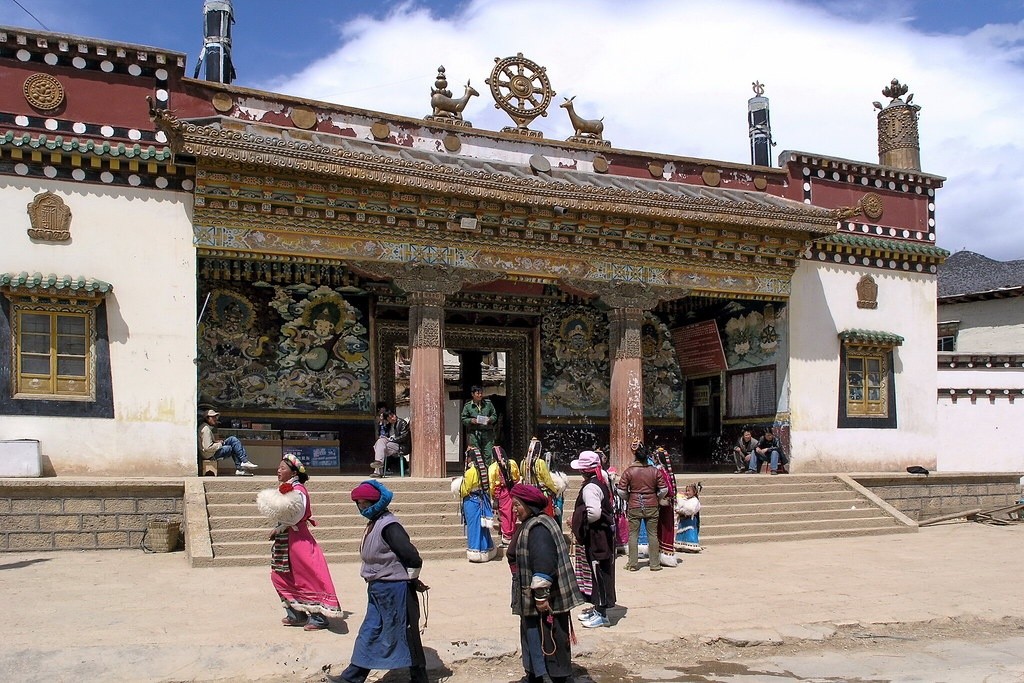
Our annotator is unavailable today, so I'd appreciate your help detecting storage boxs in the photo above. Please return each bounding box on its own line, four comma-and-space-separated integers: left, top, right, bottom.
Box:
0, 440, 43, 478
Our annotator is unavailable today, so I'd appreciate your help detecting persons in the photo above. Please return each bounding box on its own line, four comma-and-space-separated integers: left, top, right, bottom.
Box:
617, 437, 677, 571
461, 385, 497, 467
451, 444, 497, 562
566, 451, 616, 627
733, 430, 758, 474
325, 479, 430, 683
487, 446, 520, 547
541, 452, 569, 526
257, 453, 343, 631
369, 402, 412, 479
745, 426, 788, 475
505, 483, 586, 683
198, 410, 258, 475
520, 436, 557, 516
673, 483, 702, 553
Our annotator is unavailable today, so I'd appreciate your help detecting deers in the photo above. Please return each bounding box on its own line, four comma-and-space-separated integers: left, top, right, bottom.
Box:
559, 96, 604, 139
431, 79, 480, 120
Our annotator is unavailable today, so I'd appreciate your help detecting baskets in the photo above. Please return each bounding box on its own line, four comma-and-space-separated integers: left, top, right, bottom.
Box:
145, 520, 182, 553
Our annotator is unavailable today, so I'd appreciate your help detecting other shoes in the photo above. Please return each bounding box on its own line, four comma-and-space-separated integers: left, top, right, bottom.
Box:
324, 674, 353, 683
623, 563, 639, 572
235, 470, 254, 476
733, 470, 743, 474
771, 470, 778, 475
369, 472, 383, 478
509, 676, 544, 683
303, 623, 324, 631
650, 566, 663, 571
241, 461, 258, 469
745, 469, 757, 474
281, 616, 308, 627
370, 460, 385, 469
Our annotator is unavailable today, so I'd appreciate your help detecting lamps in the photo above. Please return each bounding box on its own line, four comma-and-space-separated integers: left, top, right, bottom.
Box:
461, 217, 477, 229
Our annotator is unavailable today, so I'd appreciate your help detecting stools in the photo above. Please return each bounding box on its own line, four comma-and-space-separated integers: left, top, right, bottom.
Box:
202, 460, 218, 476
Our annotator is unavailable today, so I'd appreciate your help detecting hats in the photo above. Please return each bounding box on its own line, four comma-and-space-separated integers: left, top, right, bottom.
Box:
350, 483, 382, 502
202, 409, 220, 417
570, 450, 601, 470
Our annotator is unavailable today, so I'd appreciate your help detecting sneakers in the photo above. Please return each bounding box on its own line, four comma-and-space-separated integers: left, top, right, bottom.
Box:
581, 609, 611, 628
578, 606, 601, 621
